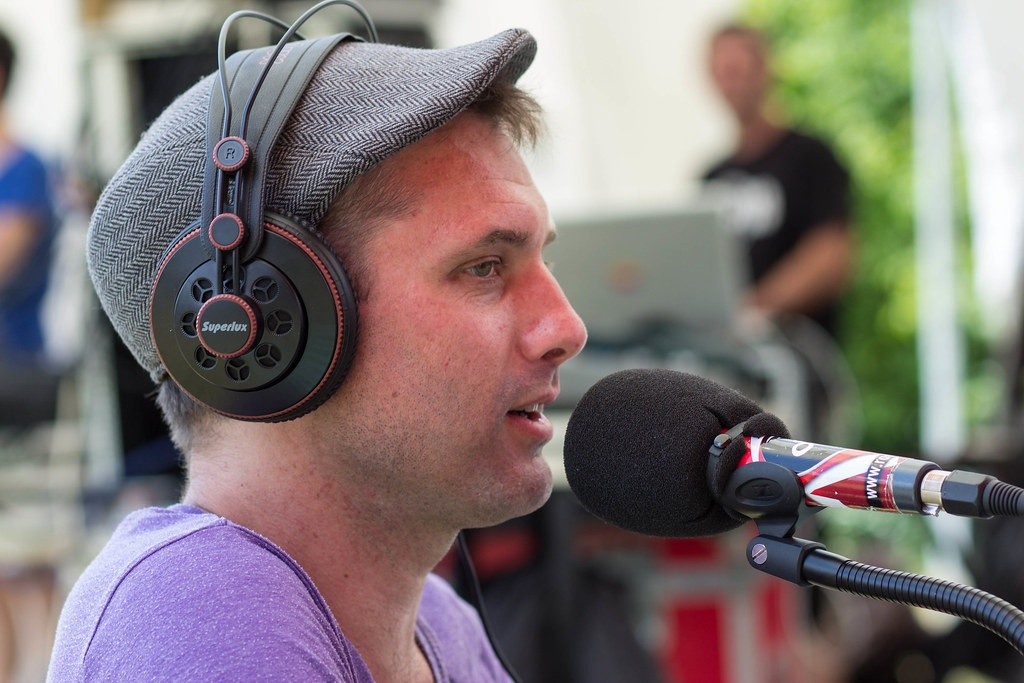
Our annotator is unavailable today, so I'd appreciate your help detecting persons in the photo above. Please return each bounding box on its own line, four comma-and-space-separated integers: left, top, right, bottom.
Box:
0, 33, 56, 438
45, 0, 588, 683
702, 23, 861, 635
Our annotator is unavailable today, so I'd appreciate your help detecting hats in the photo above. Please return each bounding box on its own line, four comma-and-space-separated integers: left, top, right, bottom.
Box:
86, 27, 549, 384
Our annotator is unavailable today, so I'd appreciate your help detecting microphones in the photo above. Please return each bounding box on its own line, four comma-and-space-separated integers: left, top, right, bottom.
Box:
564, 368, 1024, 539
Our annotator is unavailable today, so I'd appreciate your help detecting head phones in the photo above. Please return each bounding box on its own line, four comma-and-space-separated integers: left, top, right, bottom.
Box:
149, 0, 383, 423
